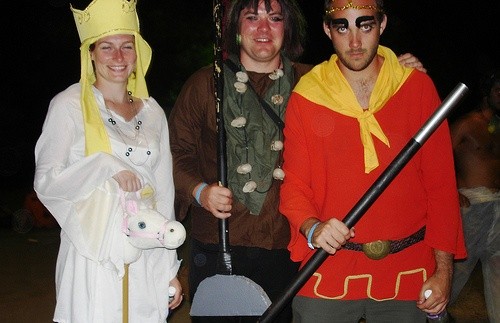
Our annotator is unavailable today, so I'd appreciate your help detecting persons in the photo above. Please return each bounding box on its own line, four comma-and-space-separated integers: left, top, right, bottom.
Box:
34, 0, 183, 322
279, 0, 468, 322
445, 72, 500, 323
167, 0, 427, 323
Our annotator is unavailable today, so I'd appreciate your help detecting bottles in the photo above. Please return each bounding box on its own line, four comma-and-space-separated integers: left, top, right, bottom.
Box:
169, 287, 175, 312
424, 290, 449, 323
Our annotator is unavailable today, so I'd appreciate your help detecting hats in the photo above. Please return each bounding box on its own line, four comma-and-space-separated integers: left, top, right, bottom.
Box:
70, 0, 152, 156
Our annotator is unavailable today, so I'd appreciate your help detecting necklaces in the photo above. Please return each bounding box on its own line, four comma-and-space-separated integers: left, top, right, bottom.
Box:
106, 90, 151, 166
232, 69, 284, 194
488, 113, 496, 135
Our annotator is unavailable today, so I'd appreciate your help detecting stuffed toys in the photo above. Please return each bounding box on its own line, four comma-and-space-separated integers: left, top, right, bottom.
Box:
108, 182, 187, 277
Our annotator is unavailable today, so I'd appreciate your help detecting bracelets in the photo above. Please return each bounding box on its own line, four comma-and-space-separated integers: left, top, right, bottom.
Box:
196, 183, 207, 205
308, 222, 320, 249
